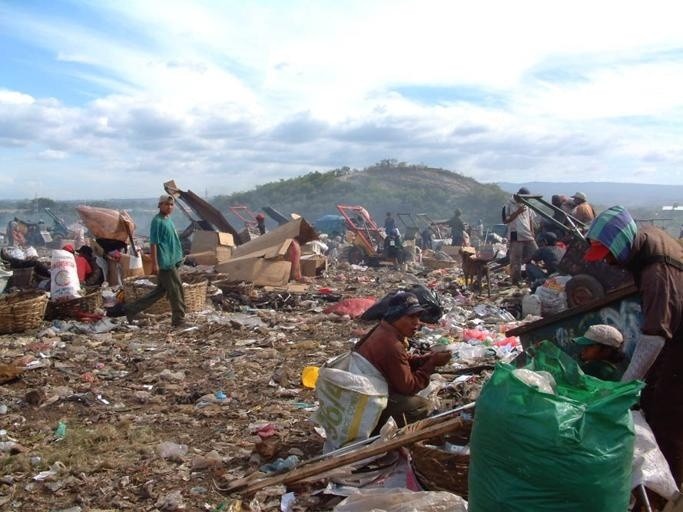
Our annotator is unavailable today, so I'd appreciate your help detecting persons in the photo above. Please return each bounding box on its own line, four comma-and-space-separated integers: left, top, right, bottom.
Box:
570, 325, 629, 381
543, 192, 594, 251
122, 194, 196, 329
469, 220, 483, 241
501, 187, 541, 286
381, 230, 404, 271
62, 240, 104, 292
524, 231, 568, 292
283, 238, 300, 280
384, 212, 397, 234
583, 205, 683, 511
446, 208, 467, 246
4, 216, 89, 251
357, 293, 452, 438
421, 222, 435, 251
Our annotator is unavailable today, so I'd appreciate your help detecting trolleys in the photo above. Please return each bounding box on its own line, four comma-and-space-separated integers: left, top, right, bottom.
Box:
336, 205, 385, 265
513, 194, 628, 309
44, 207, 71, 240
398, 212, 449, 240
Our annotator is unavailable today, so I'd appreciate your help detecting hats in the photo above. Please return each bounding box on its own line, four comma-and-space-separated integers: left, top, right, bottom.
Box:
158, 195, 174, 208
571, 192, 586, 201
385, 293, 425, 318
573, 323, 623, 348
583, 237, 610, 262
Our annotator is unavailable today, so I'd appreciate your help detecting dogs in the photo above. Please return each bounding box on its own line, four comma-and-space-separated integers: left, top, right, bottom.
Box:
457, 247, 499, 299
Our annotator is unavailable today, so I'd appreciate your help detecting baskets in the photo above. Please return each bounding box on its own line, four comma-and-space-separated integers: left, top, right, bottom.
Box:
412, 419, 471, 499
1, 290, 49, 333
52, 288, 104, 321
123, 274, 208, 314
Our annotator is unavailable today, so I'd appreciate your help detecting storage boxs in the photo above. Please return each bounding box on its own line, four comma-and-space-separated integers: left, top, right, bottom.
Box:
443, 246, 475, 267
192, 231, 233, 262
